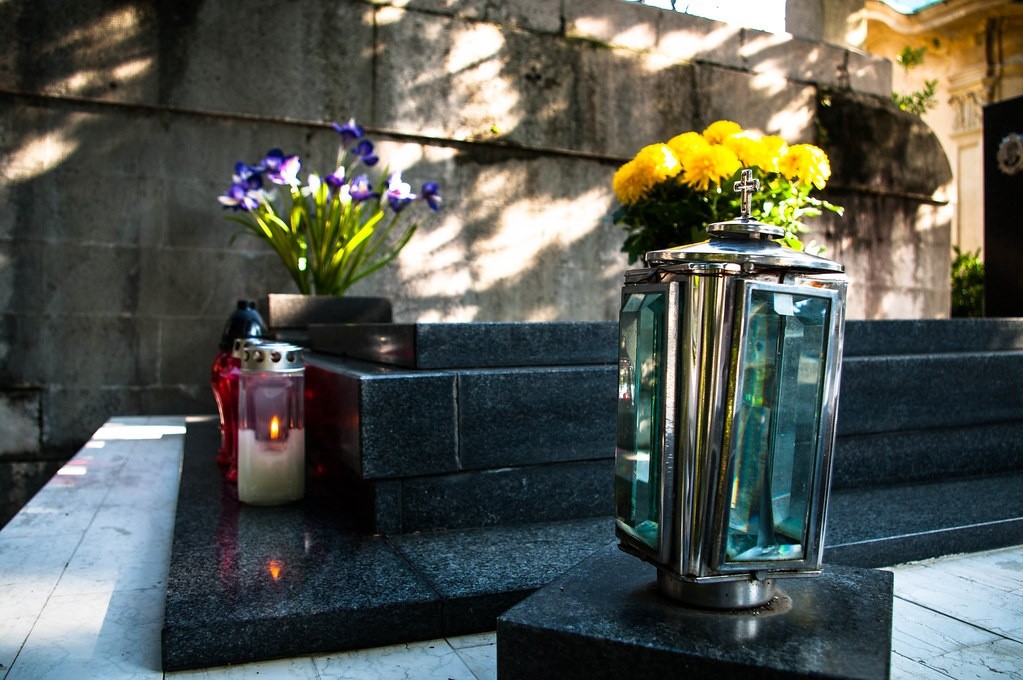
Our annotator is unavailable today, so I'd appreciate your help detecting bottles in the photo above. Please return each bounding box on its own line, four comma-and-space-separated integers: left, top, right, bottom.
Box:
212, 299, 307, 505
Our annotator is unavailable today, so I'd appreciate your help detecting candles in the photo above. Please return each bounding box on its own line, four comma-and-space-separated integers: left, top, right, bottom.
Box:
239, 417, 304, 505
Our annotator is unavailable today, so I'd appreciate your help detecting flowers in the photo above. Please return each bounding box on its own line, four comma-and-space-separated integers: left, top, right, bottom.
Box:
217, 117, 443, 296
610, 120, 844, 266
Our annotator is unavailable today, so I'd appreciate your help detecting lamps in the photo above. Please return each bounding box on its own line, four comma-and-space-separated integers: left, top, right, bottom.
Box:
612, 168, 851, 602
